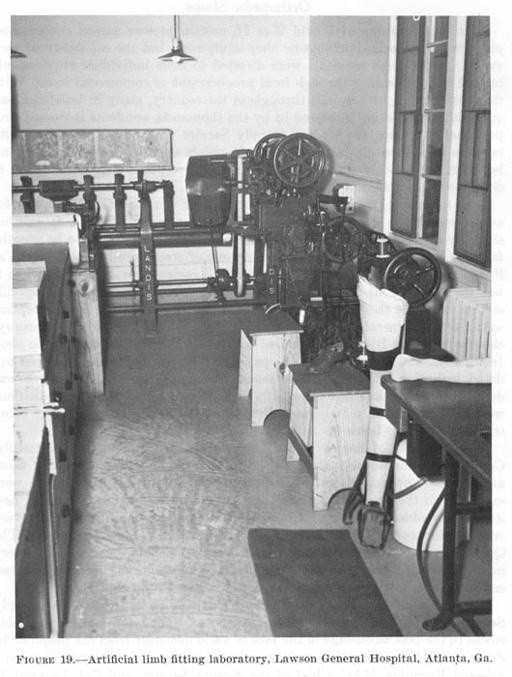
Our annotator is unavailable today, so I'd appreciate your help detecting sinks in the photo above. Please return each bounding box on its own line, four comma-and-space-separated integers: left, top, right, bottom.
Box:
478, 429, 491, 445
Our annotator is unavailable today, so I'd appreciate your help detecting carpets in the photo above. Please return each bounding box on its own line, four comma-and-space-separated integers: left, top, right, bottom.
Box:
249, 525, 401, 637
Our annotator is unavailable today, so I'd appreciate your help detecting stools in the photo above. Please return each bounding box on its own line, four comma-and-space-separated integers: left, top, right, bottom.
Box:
235, 304, 373, 513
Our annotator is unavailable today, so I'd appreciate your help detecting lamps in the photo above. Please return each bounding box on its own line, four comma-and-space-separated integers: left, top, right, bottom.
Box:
159, 15, 195, 64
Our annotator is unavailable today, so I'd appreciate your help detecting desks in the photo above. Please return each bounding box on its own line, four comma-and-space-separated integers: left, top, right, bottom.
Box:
379, 371, 492, 638
